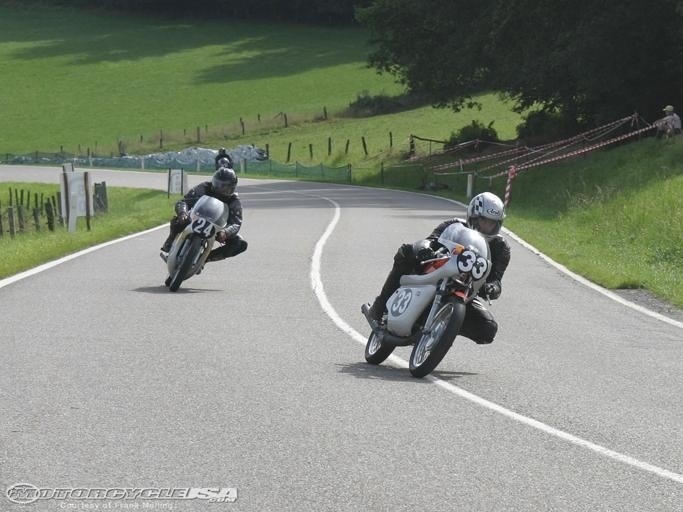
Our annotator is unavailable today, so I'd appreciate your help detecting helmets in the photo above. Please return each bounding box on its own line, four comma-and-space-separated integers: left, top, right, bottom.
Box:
467, 191, 505, 242
211, 167, 238, 200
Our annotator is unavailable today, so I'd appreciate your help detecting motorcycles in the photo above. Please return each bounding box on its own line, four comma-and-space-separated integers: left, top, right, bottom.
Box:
361, 223, 492, 378
160, 157, 229, 292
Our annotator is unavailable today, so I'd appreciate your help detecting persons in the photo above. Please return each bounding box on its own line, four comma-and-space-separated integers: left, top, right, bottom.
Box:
215, 148, 234, 171
162, 166, 249, 276
367, 192, 511, 344
654, 105, 682, 140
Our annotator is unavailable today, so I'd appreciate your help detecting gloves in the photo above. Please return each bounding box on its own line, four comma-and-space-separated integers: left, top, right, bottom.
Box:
174, 211, 189, 224
416, 247, 435, 261
216, 229, 227, 243
481, 282, 500, 299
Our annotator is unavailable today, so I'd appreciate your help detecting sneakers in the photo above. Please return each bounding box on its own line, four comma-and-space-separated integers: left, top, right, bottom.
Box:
163, 238, 172, 252
368, 296, 384, 321
196, 253, 208, 275
